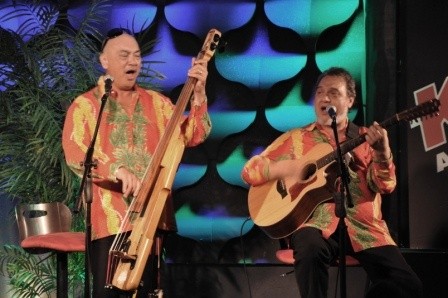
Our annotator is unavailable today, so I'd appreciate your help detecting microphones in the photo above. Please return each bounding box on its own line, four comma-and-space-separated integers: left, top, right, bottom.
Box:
104, 74, 113, 96
326, 105, 337, 117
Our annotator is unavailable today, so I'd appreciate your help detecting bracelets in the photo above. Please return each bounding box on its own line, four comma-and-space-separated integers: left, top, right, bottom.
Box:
374, 154, 393, 163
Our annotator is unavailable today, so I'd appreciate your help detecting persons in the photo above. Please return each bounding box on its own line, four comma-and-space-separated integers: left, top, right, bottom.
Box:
62, 28, 212, 298
242, 66, 424, 298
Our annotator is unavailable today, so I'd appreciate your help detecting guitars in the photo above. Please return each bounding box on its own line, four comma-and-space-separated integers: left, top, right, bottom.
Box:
247, 98, 439, 240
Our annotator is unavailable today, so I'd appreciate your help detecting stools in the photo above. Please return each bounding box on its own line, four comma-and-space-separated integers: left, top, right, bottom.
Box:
274, 236, 370, 298
15, 202, 86, 298
